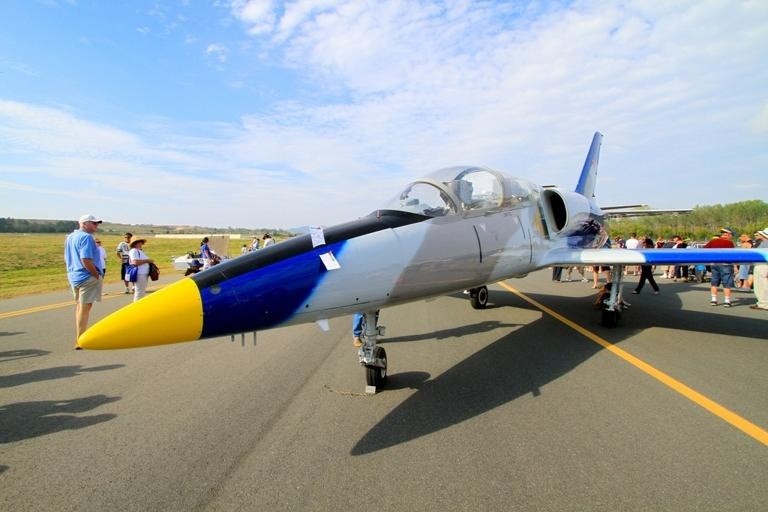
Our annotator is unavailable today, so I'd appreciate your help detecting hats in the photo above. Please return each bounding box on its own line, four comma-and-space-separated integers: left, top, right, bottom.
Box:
757, 228, 767, 238
78, 215, 101, 223
187, 260, 203, 267
129, 236, 146, 246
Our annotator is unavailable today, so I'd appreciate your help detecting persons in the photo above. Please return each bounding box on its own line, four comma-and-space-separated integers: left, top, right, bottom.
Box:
64, 214, 104, 351
240, 233, 275, 255
552, 227, 767, 313
116, 232, 137, 294
94, 237, 108, 296
184, 238, 226, 276
128, 235, 155, 304
352, 311, 380, 347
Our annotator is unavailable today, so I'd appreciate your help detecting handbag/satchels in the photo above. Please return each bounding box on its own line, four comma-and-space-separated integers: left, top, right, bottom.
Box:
149, 262, 159, 281
125, 265, 137, 282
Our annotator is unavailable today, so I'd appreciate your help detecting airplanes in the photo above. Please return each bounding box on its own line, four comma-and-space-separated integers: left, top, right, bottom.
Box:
76, 132, 768, 386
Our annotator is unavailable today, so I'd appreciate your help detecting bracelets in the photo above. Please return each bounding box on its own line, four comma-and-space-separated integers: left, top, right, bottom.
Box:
143, 259, 145, 263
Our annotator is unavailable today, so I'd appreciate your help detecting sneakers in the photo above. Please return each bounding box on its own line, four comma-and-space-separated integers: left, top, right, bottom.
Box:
353, 339, 362, 347
124, 288, 128, 293
552, 269, 766, 309
